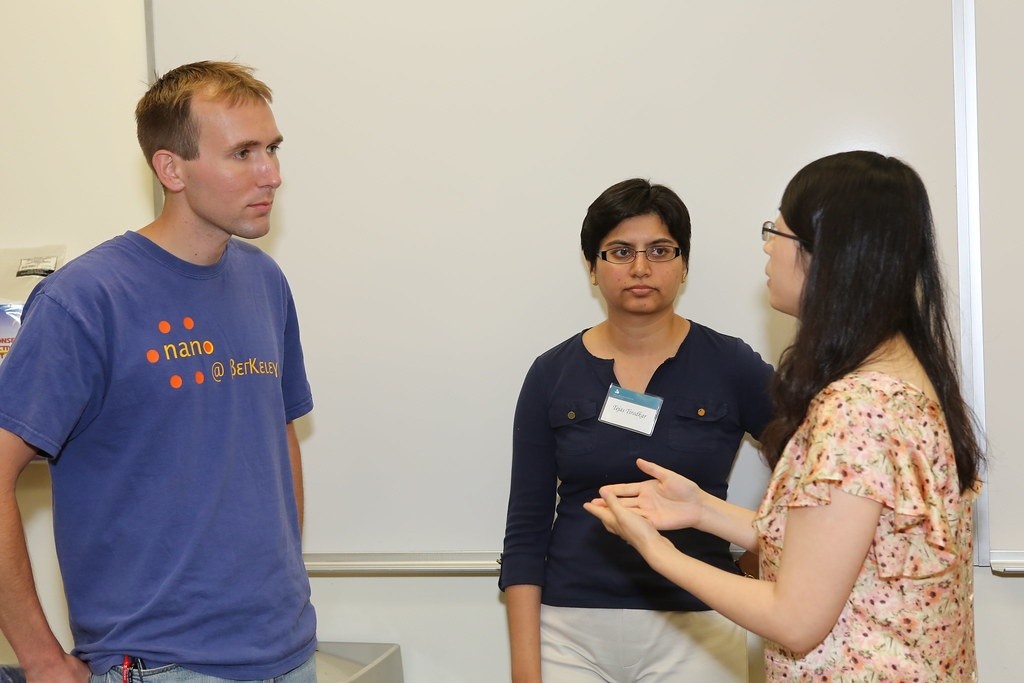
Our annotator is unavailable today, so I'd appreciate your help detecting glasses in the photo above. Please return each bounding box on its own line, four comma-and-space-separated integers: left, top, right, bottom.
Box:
593, 245, 681, 264
761, 220, 800, 242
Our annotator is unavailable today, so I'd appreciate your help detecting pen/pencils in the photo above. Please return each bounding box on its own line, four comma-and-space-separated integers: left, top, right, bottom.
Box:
137, 658, 144, 683
123, 656, 135, 683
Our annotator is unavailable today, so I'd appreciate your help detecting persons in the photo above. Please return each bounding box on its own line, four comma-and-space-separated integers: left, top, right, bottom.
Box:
581, 149, 985, 683
496, 177, 817, 683
0, 59, 316, 683
